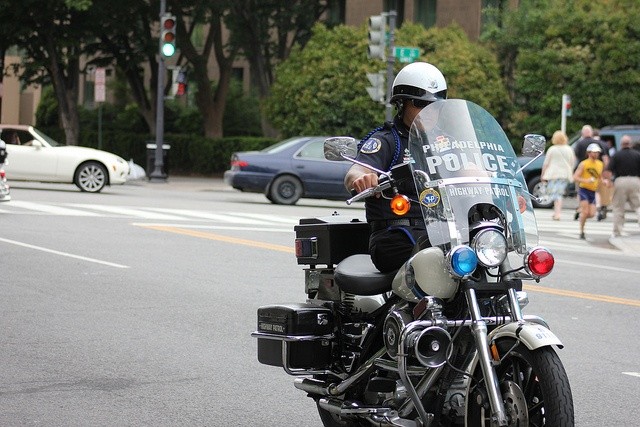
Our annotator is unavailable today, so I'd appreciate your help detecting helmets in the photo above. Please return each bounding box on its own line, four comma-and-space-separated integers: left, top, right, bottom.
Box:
389, 61, 448, 121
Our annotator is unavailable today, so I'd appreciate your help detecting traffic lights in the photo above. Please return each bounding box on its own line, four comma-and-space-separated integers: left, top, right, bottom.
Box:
365, 73, 385, 102
367, 16, 385, 61
172, 67, 187, 99
565, 95, 572, 116
161, 16, 177, 63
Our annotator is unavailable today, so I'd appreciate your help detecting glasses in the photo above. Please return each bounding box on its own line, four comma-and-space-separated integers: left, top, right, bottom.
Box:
406, 97, 433, 109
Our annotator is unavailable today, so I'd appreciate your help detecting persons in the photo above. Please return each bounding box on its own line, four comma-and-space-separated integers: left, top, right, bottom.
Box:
343, 61, 498, 273
602, 133, 640, 238
539, 129, 578, 221
573, 124, 611, 220
574, 142, 610, 240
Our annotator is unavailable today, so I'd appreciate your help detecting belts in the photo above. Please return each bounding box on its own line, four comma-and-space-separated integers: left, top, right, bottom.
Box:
370, 217, 425, 230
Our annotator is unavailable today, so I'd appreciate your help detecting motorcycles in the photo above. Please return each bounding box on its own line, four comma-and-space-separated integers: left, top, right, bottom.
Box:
250, 98, 575, 427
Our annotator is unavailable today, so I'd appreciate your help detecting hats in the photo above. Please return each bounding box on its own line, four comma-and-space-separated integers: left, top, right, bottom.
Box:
586, 143, 602, 152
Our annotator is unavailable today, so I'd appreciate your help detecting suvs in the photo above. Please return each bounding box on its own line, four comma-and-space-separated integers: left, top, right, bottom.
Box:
518, 126, 640, 208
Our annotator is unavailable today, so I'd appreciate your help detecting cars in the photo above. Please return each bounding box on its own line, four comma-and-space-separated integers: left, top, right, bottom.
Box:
0, 124, 129, 193
224, 137, 365, 204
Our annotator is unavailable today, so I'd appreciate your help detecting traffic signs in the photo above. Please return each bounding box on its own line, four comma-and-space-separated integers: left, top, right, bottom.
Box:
394, 48, 419, 63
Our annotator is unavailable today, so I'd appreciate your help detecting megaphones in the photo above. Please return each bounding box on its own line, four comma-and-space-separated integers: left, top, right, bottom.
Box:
413, 325, 454, 369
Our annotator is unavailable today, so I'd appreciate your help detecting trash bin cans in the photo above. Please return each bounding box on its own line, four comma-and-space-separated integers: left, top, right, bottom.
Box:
144, 139, 171, 182
